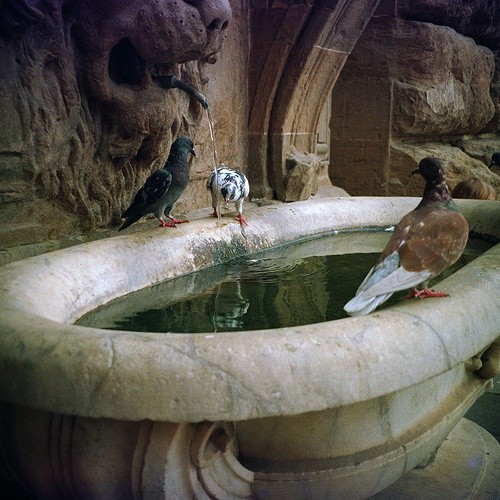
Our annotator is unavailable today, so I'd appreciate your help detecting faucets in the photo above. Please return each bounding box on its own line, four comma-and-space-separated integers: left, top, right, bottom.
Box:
155, 73, 209, 109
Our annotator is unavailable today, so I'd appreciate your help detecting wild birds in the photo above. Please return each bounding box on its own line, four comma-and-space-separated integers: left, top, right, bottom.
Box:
206, 168, 249, 226
119, 137, 196, 232
344, 157, 469, 316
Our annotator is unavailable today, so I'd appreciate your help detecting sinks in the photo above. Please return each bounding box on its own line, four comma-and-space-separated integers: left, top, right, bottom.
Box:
0, 195, 500, 499
